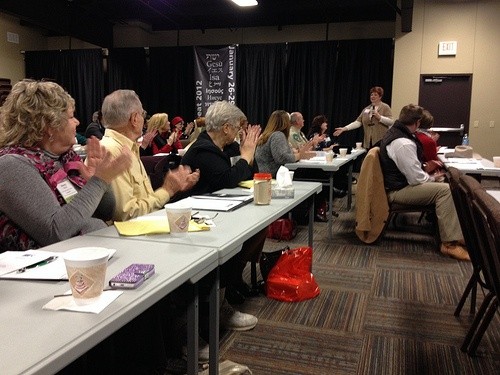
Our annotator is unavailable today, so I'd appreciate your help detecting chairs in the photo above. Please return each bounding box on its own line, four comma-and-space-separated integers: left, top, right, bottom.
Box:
446, 168, 500, 355
373, 147, 442, 254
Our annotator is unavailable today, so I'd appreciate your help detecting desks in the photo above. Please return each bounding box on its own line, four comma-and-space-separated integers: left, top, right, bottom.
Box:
438, 146, 500, 186
86, 180, 321, 375
0, 236, 220, 375
284, 148, 365, 242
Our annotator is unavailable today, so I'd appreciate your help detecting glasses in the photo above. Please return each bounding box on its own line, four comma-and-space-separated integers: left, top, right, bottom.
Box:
229, 124, 242, 130
177, 121, 184, 125
137, 110, 147, 119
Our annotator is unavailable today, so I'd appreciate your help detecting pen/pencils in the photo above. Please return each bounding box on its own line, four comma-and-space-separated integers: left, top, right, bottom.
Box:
15, 256, 59, 273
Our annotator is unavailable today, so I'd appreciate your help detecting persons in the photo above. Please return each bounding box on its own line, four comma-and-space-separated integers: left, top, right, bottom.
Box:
254, 110, 339, 223
306, 115, 358, 185
287, 112, 347, 199
139, 110, 206, 156
333, 86, 394, 154
75, 110, 105, 146
379, 104, 471, 261
84, 89, 258, 364
179, 100, 262, 296
0, 78, 132, 253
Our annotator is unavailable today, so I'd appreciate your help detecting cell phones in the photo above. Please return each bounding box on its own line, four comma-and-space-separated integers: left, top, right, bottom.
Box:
109, 264, 155, 289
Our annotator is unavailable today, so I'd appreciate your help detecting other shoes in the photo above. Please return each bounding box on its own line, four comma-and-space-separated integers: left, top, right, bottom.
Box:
317, 213, 328, 222
333, 187, 346, 198
441, 243, 471, 262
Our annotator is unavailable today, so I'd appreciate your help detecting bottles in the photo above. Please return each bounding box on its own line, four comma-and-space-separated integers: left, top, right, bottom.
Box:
253, 173, 272, 206
462, 134, 468, 145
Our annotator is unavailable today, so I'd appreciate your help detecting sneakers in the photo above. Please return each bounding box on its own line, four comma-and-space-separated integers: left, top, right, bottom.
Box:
182, 332, 211, 364
219, 302, 258, 332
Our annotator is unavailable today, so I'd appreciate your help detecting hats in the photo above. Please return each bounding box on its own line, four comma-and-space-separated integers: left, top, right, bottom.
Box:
171, 116, 183, 126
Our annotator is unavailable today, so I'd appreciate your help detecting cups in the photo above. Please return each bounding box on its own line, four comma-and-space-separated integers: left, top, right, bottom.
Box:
339, 148, 348, 157
326, 153, 333, 161
493, 156, 500, 168
63, 247, 110, 303
178, 149, 185, 156
164, 203, 192, 238
356, 143, 362, 149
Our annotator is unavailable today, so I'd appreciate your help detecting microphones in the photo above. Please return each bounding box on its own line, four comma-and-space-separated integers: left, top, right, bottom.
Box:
372, 103, 375, 117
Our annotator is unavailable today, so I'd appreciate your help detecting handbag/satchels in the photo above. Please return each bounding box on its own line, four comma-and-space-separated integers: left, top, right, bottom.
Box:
260, 246, 320, 303
265, 219, 299, 243
